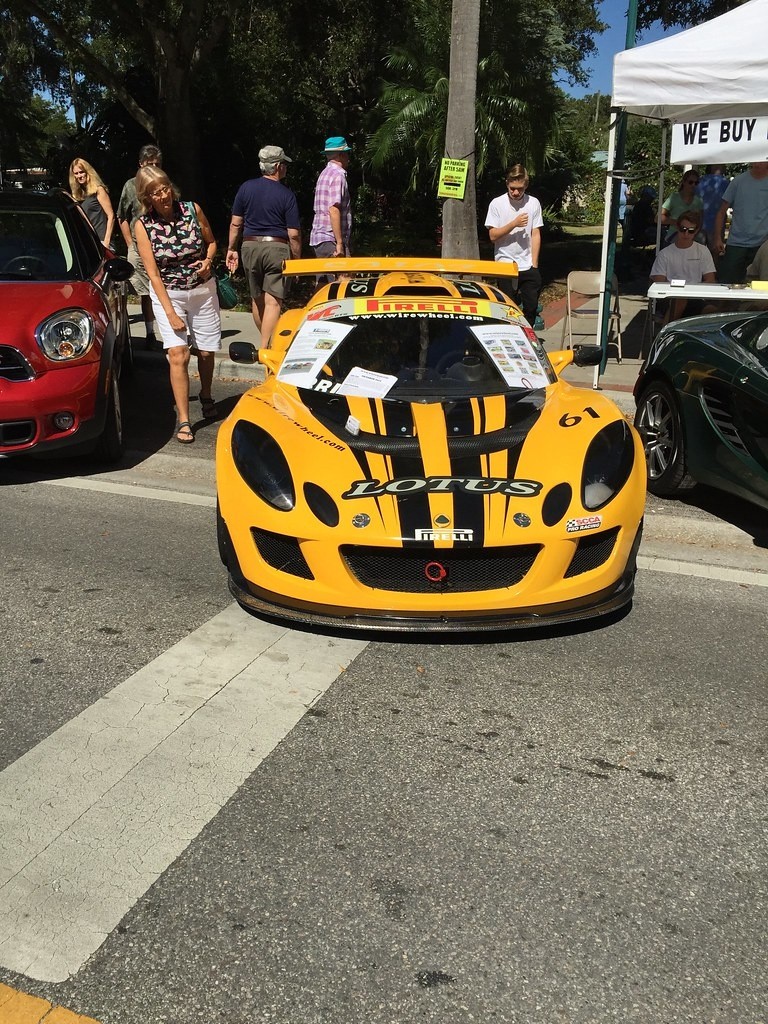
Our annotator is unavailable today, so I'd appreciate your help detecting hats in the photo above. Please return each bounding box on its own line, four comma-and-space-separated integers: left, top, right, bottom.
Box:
642, 186, 657, 199
258, 145, 292, 162
324, 136, 352, 151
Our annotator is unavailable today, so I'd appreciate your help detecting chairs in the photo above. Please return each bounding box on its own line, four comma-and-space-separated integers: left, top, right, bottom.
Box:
622, 208, 649, 252
362, 345, 403, 375
562, 270, 623, 364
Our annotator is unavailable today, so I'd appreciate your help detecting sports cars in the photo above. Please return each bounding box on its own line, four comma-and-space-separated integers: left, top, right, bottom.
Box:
632, 309, 768, 519
215, 258, 647, 631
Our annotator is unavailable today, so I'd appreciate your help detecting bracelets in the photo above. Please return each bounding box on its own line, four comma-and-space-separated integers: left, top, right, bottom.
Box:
206, 257, 213, 263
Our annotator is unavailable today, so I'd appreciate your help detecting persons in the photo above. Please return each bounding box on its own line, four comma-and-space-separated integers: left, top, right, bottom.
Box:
134, 163, 222, 443
619, 163, 733, 286
68, 158, 115, 249
746, 240, 768, 282
308, 137, 352, 294
118, 145, 199, 355
712, 161, 768, 312
486, 164, 544, 329
226, 146, 302, 350
650, 208, 720, 325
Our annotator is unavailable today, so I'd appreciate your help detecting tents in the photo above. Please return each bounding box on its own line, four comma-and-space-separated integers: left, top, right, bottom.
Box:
593, 0, 768, 390
668, 116, 768, 173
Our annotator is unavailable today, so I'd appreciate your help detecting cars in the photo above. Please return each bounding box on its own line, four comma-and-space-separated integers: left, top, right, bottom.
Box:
0, 180, 136, 467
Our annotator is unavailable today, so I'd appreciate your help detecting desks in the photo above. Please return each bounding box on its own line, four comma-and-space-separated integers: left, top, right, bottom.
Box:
639, 282, 768, 358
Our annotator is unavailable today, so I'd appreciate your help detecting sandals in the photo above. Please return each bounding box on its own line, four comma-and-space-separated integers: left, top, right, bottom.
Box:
177, 422, 194, 443
198, 392, 218, 418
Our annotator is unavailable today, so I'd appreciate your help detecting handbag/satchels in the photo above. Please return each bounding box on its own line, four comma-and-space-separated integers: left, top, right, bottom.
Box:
213, 271, 239, 310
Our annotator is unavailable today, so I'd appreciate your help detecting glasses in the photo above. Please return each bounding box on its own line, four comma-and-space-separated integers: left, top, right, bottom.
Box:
144, 162, 160, 168
683, 179, 700, 186
678, 225, 698, 234
149, 186, 168, 196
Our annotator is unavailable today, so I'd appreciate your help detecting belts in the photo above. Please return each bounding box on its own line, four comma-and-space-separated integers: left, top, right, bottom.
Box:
243, 236, 290, 244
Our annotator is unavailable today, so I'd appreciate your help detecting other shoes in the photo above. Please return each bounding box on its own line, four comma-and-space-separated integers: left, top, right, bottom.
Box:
146, 329, 160, 350
